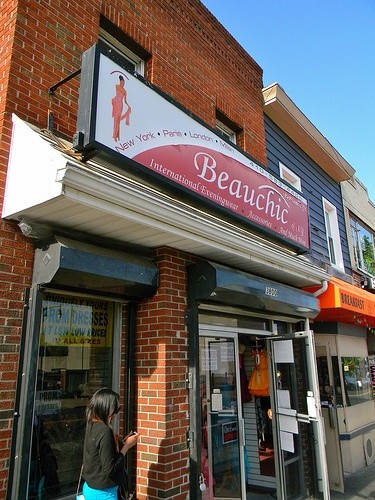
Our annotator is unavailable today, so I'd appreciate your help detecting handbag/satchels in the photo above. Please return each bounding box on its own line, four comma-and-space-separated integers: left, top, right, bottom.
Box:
76, 495, 85, 500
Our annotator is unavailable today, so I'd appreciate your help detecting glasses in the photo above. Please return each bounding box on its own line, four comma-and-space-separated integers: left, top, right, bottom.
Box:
115, 407, 121, 414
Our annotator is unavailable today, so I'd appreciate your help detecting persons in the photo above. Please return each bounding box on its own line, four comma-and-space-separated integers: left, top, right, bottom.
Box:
83, 388, 140, 500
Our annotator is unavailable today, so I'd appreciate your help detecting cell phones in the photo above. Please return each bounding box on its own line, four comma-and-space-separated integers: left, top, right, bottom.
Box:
123, 432, 136, 444
